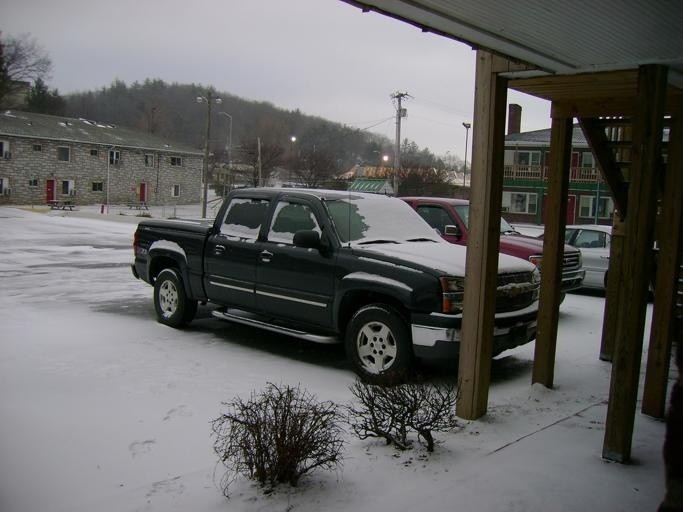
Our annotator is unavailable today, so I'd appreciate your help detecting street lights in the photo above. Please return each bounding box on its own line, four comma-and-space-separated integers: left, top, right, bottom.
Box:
460, 120, 471, 188
216, 109, 233, 164
195, 89, 223, 223
288, 135, 296, 180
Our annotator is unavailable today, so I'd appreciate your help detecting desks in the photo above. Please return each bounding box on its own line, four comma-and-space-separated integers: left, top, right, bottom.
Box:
49, 199, 72, 211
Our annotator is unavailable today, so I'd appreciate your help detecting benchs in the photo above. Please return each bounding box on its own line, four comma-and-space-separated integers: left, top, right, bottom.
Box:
47, 204, 74, 211
